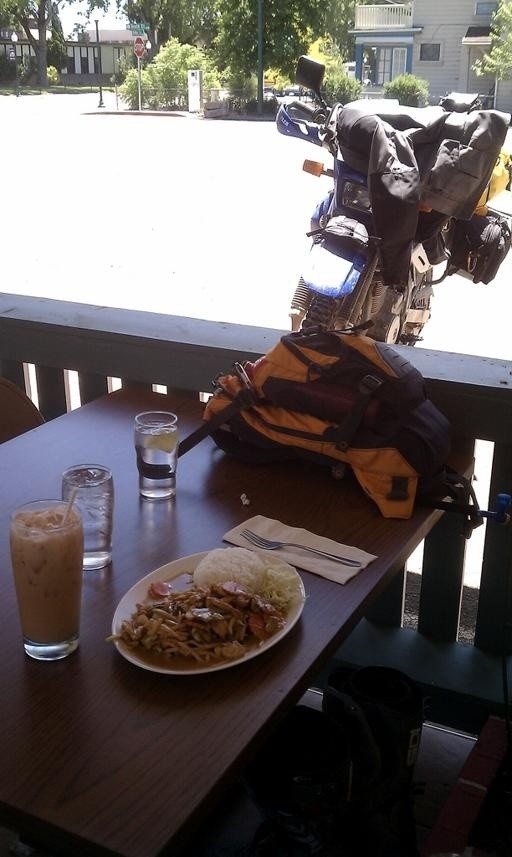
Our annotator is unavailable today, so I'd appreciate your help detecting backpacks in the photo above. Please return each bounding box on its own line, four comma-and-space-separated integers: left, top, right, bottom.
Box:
200, 320, 454, 521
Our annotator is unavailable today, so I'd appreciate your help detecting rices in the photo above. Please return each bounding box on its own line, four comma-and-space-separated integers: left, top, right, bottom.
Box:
193, 547, 265, 590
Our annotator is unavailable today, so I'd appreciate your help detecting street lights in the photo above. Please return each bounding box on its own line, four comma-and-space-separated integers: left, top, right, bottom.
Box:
11, 31, 23, 96
91, 4, 108, 108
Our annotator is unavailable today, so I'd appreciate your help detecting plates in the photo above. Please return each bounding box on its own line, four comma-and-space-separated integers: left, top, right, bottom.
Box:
111, 547, 308, 677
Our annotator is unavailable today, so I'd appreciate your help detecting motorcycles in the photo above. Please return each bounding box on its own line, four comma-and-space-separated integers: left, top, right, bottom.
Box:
272, 53, 512, 343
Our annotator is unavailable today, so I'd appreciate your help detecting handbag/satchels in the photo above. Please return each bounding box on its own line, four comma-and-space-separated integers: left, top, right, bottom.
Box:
451, 208, 511, 285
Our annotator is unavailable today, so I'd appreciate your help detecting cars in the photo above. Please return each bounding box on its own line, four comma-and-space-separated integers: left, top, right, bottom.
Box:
271, 79, 312, 96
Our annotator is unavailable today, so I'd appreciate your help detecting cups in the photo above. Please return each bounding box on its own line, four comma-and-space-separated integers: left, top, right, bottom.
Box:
60, 463, 117, 572
6, 496, 87, 663
132, 409, 179, 500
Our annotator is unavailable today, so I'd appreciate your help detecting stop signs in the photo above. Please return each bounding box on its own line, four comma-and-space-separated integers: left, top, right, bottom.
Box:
134, 36, 145, 59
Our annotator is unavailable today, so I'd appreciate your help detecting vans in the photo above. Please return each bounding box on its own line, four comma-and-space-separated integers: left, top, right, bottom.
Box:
342, 62, 371, 87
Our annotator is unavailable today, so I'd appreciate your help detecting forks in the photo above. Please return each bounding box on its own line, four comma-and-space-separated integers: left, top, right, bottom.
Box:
238, 525, 363, 570
